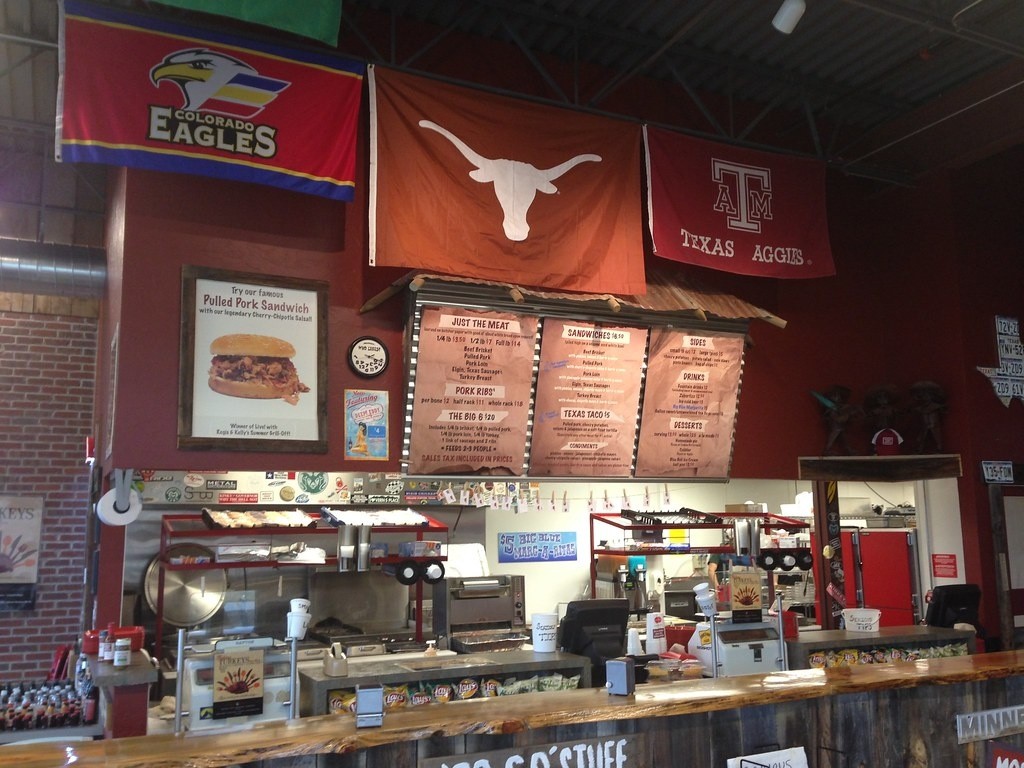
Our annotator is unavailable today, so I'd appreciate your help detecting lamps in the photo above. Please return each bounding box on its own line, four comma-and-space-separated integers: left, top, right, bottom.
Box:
771, 0, 806, 35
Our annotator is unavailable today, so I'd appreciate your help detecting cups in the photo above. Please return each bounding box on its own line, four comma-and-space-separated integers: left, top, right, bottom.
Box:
287, 613, 311, 640
627, 613, 667, 655
695, 592, 716, 616
290, 599, 310, 613
532, 614, 557, 653
693, 583, 709, 597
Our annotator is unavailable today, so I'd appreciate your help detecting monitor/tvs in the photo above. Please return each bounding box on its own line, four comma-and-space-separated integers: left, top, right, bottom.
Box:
559, 598, 630, 656
928, 583, 982, 631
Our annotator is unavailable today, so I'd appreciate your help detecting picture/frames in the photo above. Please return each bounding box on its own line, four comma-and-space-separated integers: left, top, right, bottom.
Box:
176, 263, 328, 453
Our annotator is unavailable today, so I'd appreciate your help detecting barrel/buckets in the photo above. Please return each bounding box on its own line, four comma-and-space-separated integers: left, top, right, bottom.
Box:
841, 608, 881, 632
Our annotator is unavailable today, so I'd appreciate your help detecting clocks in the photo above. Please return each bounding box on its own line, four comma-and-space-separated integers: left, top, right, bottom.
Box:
348, 336, 388, 378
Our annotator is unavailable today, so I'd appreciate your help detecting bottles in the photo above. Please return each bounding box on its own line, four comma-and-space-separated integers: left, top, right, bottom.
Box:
0, 652, 97, 727
99, 631, 108, 661
113, 639, 131, 668
103, 623, 115, 664
